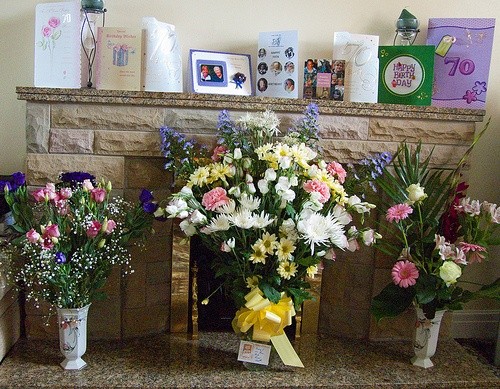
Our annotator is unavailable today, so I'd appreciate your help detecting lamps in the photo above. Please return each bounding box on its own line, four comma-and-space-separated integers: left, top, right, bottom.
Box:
392, 1, 422, 47
79, 0, 107, 89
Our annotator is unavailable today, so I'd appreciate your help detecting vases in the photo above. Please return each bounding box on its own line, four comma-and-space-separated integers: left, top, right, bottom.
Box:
236, 325, 279, 372
406, 296, 450, 367
52, 301, 92, 370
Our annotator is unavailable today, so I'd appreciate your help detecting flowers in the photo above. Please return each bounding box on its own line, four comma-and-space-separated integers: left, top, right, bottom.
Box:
357, 113, 499, 327
141, 98, 390, 343
0, 170, 171, 328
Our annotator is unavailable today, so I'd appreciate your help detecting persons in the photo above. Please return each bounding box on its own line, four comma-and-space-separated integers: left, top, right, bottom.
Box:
199, 45, 345, 101
